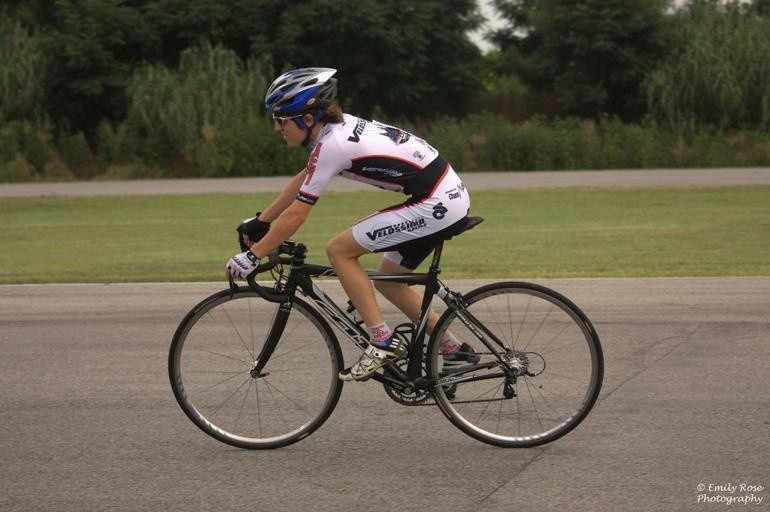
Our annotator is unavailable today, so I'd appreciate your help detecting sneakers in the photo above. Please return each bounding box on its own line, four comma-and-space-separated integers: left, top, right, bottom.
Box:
442, 343, 476, 392
339, 334, 408, 381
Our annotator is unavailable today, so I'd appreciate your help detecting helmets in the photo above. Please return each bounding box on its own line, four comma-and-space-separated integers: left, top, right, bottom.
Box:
265, 67, 338, 112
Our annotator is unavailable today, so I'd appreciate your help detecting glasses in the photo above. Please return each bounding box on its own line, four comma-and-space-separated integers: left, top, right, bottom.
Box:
272, 113, 303, 125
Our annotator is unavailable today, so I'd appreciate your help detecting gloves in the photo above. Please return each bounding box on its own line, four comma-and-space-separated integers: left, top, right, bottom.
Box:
225, 250, 260, 281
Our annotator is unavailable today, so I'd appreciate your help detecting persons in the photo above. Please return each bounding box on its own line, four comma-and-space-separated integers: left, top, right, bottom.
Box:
226, 67, 471, 392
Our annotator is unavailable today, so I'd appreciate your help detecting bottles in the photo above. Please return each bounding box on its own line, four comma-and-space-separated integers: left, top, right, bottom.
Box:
346, 300, 373, 335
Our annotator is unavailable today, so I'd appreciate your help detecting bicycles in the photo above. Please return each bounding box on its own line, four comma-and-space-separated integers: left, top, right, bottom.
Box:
168, 216, 604, 449
